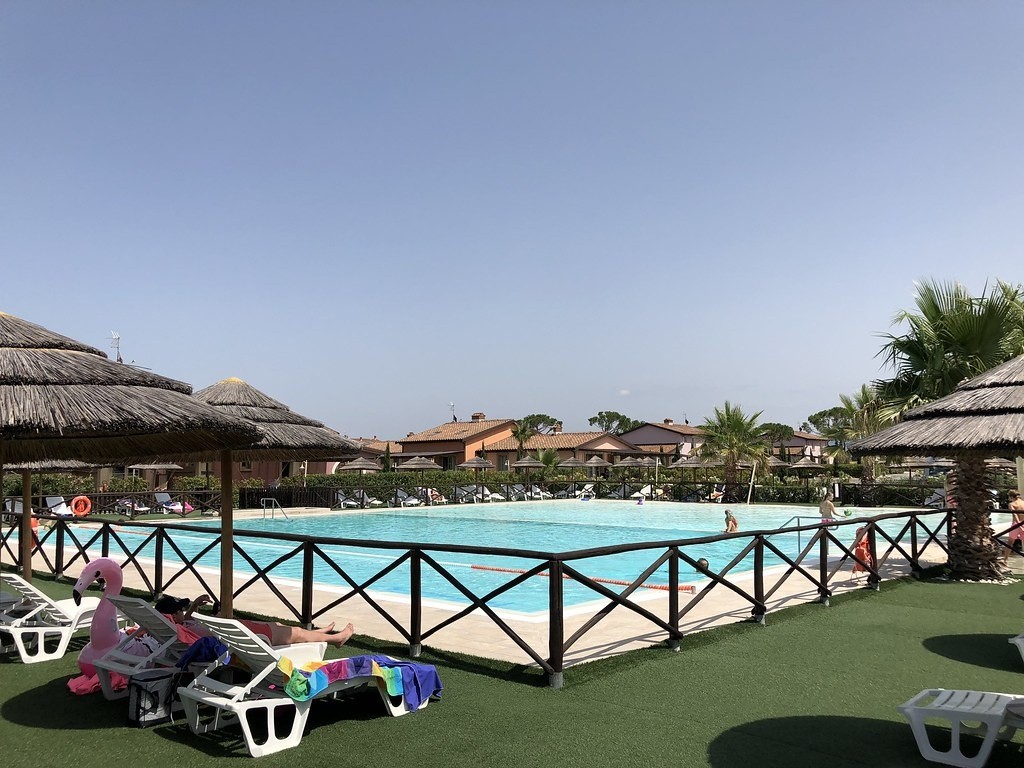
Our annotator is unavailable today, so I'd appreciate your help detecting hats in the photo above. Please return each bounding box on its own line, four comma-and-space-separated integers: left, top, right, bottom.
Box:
155, 596, 190, 614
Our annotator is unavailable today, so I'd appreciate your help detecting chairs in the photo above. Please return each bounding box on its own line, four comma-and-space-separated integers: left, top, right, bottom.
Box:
630, 484, 657, 500
45, 497, 77, 518
95, 595, 329, 712
705, 484, 726, 503
116, 499, 151, 516
3, 498, 25, 523
336, 480, 597, 509
0, 573, 130, 664
176, 611, 435, 758
154, 493, 183, 514
893, 688, 1024, 768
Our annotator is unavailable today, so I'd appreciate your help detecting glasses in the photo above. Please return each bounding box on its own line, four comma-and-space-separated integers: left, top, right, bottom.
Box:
178, 607, 185, 613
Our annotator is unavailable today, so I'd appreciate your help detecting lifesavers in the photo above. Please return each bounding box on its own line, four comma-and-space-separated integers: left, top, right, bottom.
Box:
431, 494, 439, 501
70, 495, 92, 516
853, 527, 870, 573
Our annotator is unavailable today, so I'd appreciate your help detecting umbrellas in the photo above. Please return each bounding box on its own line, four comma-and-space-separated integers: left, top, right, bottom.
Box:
0, 311, 363, 605
457, 456, 496, 492
790, 457, 826, 497
736, 453, 792, 490
339, 457, 383, 499
511, 455, 546, 490
557, 457, 587, 495
668, 456, 727, 490
396, 457, 443, 494
586, 456, 613, 480
612, 456, 663, 489
846, 354, 1024, 453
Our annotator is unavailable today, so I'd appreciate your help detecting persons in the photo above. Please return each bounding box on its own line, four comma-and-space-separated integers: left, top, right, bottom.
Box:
722, 510, 739, 533
819, 493, 847, 526
99, 480, 109, 493
944, 473, 955, 509
696, 558, 708, 573
429, 489, 443, 501
154, 594, 354, 648
1001, 490, 1024, 565
605, 469, 611, 480
